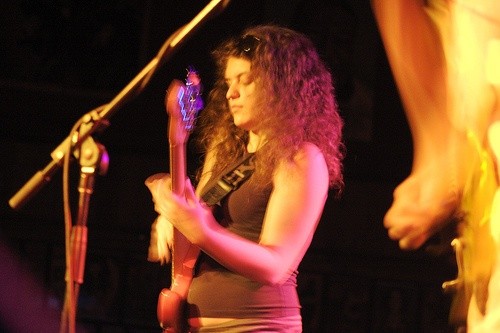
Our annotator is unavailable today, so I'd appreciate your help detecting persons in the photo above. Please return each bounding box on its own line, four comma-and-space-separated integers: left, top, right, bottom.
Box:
144, 24, 344, 333
375, 0, 500, 333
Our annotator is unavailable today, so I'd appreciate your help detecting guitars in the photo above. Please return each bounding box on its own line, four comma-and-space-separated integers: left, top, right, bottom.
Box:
157, 67, 202, 332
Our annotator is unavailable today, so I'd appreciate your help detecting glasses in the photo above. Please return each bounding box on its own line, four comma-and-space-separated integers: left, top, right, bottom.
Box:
224, 33, 260, 55
